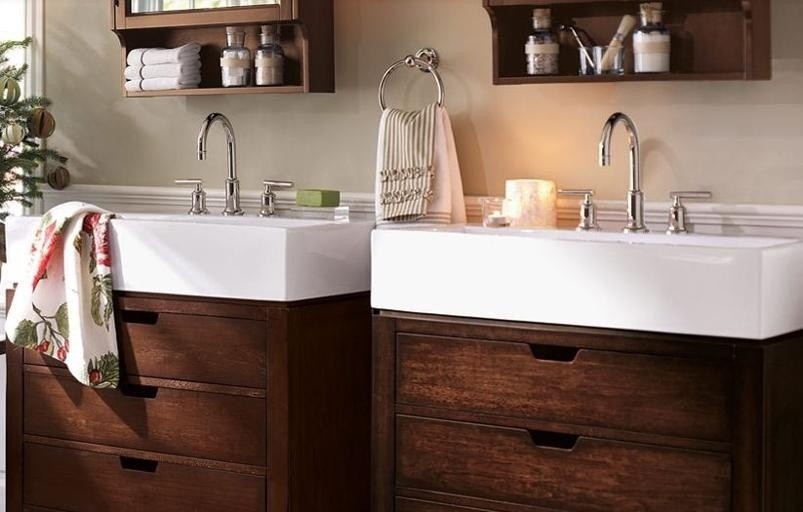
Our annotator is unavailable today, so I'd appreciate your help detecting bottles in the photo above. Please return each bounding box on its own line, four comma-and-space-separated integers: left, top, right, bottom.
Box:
256, 22, 289, 85
633, 2, 672, 74
526, 5, 562, 76
220, 24, 252, 87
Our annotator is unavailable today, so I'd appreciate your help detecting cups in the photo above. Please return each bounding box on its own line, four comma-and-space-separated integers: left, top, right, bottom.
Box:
593, 45, 624, 77
480, 196, 512, 229
578, 44, 594, 77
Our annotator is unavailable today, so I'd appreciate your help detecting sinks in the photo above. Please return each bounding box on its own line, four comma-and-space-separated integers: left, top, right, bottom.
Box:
367, 217, 801, 343
10, 209, 366, 302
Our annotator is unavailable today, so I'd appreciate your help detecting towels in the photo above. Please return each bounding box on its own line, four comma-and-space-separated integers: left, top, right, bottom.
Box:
375, 103, 439, 223
504, 178, 558, 230
122, 41, 204, 92
373, 104, 467, 226
4, 201, 123, 391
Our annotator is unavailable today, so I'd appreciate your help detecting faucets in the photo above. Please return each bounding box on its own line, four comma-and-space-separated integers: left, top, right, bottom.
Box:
598, 111, 649, 237
195, 112, 245, 219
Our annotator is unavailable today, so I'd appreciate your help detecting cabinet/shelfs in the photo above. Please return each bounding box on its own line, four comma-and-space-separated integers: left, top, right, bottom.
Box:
482, 1, 771, 86
5, 284, 378, 512
370, 310, 803, 512
109, 0, 335, 99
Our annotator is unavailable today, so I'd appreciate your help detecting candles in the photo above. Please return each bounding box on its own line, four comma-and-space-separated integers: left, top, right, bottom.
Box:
487, 212, 509, 226
